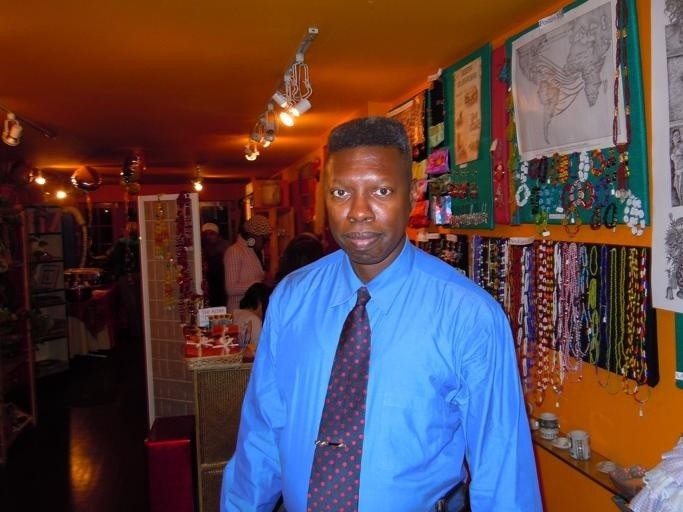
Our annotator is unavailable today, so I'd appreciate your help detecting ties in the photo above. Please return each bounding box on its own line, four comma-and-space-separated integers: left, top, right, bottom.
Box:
307, 287, 371, 511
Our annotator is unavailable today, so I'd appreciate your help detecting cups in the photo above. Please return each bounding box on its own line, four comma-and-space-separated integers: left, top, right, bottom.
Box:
538, 413, 558, 440
566, 430, 591, 461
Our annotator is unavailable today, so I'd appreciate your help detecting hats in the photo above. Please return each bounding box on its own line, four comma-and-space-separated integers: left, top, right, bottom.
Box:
245, 215, 273, 235
201, 223, 220, 234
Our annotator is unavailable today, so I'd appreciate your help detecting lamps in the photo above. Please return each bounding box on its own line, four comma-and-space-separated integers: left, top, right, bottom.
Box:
272, 63, 313, 126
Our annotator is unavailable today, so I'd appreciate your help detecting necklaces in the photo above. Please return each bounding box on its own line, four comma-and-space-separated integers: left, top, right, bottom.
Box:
611, 1, 634, 191
421, 238, 462, 273
471, 236, 648, 405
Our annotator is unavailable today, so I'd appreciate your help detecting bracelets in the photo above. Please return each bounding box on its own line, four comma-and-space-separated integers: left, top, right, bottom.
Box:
442, 166, 489, 230
513, 143, 648, 239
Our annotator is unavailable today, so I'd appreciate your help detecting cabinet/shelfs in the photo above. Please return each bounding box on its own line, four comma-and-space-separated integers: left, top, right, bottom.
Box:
0, 206, 69, 462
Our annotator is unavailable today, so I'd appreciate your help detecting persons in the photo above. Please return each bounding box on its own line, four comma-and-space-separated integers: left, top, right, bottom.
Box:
83, 220, 141, 300
216, 115, 545, 512
200, 213, 328, 349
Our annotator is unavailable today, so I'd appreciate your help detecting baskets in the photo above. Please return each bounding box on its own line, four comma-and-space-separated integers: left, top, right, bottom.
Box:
180, 350, 244, 372
